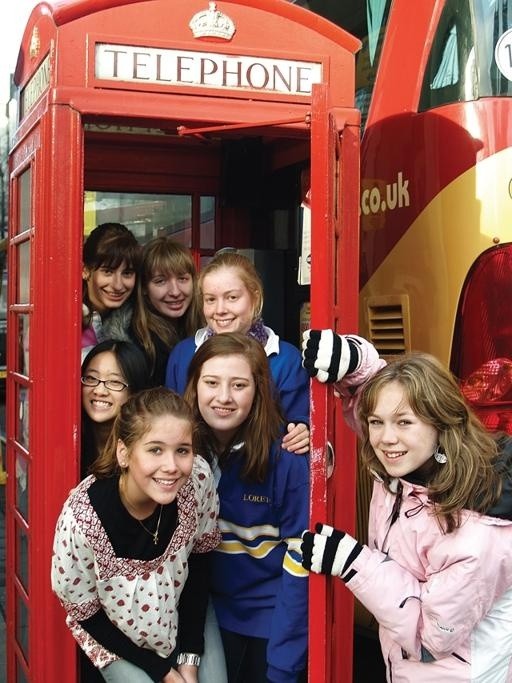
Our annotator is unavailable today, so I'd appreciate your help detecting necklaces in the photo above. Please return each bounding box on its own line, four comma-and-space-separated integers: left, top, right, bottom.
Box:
119, 485, 164, 548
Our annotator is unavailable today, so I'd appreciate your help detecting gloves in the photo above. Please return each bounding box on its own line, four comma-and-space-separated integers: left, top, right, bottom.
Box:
300, 327, 362, 385
299, 521, 363, 582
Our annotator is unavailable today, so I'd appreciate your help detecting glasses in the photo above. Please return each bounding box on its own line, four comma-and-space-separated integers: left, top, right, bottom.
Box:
77, 371, 130, 393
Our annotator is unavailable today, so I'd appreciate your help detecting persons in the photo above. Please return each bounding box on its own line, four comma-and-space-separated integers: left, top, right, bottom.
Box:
78, 335, 156, 484
130, 235, 200, 383
80, 218, 142, 343
167, 244, 312, 458
181, 331, 311, 681
295, 322, 512, 680
53, 384, 223, 682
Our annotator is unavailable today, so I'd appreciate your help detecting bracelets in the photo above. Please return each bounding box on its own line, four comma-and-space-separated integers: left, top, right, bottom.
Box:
177, 653, 202, 667
